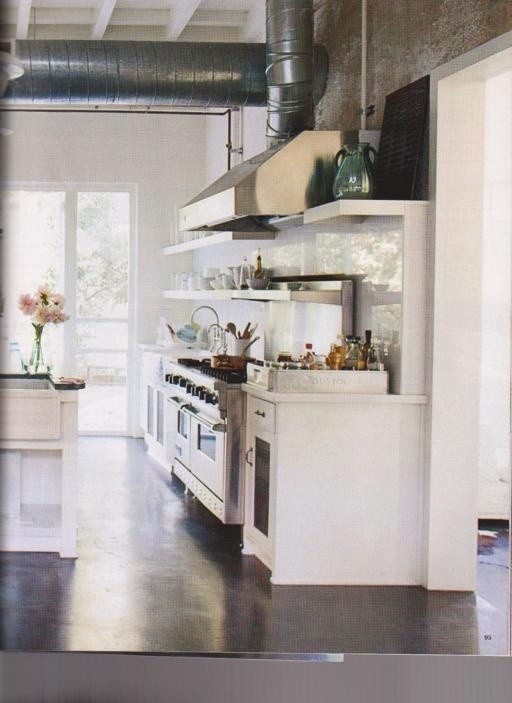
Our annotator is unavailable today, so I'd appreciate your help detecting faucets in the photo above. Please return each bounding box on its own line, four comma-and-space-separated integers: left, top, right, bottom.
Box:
207, 324, 224, 352
189, 305, 219, 352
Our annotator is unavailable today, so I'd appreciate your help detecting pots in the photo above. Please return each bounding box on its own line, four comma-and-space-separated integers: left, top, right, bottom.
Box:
210, 336, 261, 370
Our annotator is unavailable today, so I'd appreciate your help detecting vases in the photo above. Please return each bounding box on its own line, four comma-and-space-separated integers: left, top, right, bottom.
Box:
28, 336, 48, 377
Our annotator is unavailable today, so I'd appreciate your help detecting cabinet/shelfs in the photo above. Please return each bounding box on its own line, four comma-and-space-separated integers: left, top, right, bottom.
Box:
235, 382, 428, 584
161, 229, 353, 306
0, 376, 84, 559
132, 343, 174, 471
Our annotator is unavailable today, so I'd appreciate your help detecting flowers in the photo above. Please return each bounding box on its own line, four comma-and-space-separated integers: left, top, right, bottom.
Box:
17, 283, 75, 328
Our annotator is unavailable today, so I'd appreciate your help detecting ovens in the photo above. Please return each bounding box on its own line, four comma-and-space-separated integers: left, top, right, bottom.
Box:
162, 392, 243, 529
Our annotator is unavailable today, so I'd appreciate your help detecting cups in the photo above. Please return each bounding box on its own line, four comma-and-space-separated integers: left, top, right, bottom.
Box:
169, 264, 240, 291
366, 347, 384, 371
6, 342, 25, 372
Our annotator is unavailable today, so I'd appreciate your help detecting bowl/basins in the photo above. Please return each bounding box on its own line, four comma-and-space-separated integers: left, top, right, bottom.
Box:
244, 276, 271, 290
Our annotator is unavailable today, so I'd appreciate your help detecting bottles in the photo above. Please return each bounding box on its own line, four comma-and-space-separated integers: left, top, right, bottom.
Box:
239, 256, 250, 289
332, 139, 380, 199
274, 326, 373, 371
252, 256, 264, 277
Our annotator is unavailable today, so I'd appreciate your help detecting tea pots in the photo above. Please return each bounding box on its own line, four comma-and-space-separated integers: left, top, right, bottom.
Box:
162, 304, 220, 348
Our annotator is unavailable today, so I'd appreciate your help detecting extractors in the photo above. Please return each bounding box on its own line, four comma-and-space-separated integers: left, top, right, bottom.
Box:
174, 128, 360, 236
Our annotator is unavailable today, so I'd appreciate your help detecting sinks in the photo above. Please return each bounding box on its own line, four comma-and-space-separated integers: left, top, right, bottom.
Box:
152, 349, 212, 359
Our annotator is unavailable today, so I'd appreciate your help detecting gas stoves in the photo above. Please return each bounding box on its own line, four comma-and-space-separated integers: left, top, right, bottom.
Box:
161, 356, 267, 411
